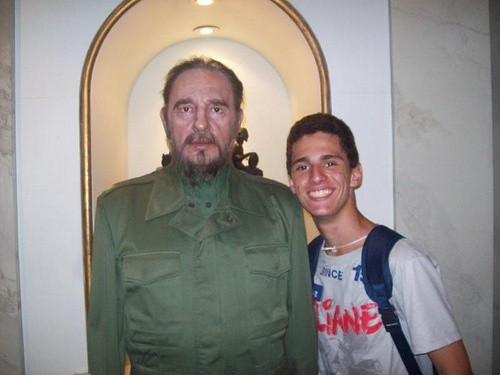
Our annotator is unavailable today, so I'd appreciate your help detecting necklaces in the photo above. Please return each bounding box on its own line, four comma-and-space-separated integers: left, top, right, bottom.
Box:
311, 230, 372, 255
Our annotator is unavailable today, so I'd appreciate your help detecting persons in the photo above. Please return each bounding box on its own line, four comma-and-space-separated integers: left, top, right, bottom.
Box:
89, 56, 319, 374
280, 112, 474, 375
